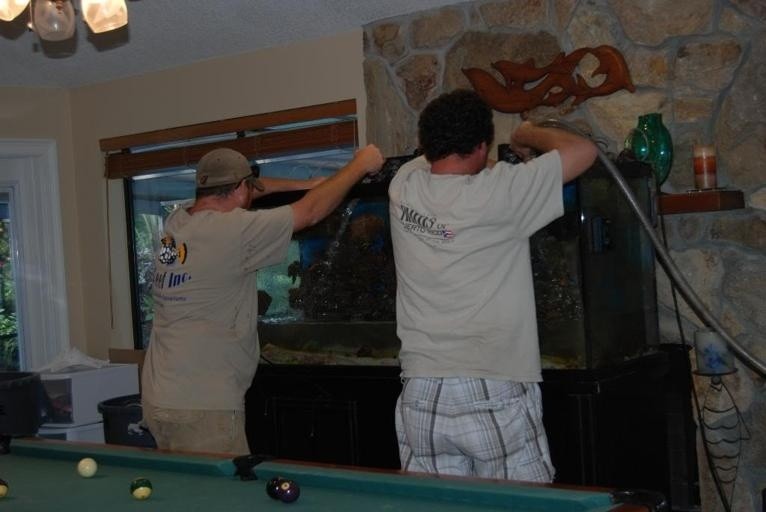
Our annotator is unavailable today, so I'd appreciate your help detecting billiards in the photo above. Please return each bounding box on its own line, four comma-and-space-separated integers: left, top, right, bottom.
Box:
265, 477, 284, 500
78, 458, 98, 478
0, 477, 8, 500
278, 480, 300, 504
130, 477, 153, 501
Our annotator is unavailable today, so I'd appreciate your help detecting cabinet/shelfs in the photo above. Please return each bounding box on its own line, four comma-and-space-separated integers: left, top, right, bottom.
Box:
247, 344, 699, 512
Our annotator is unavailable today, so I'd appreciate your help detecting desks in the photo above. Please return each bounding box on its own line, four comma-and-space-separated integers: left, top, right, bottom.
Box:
0, 436, 666, 512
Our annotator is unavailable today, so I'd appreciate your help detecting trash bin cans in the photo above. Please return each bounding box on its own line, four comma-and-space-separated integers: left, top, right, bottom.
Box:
97, 394, 158, 448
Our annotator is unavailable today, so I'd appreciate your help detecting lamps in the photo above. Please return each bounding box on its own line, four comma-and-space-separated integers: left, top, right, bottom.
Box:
0, 0, 129, 42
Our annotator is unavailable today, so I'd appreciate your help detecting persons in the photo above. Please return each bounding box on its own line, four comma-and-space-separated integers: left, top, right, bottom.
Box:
385, 87, 601, 488
137, 143, 385, 456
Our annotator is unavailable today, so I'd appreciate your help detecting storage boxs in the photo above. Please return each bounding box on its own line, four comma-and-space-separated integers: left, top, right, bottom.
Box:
36, 422, 104, 442
39, 362, 139, 428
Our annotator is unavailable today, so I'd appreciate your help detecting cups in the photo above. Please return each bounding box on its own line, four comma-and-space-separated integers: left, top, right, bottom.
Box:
695, 328, 734, 374
692, 143, 717, 190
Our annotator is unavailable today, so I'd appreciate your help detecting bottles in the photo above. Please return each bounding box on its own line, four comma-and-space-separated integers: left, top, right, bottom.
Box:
632, 111, 672, 187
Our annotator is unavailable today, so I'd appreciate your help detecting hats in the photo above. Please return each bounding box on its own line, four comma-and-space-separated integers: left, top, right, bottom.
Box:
193, 147, 268, 193
